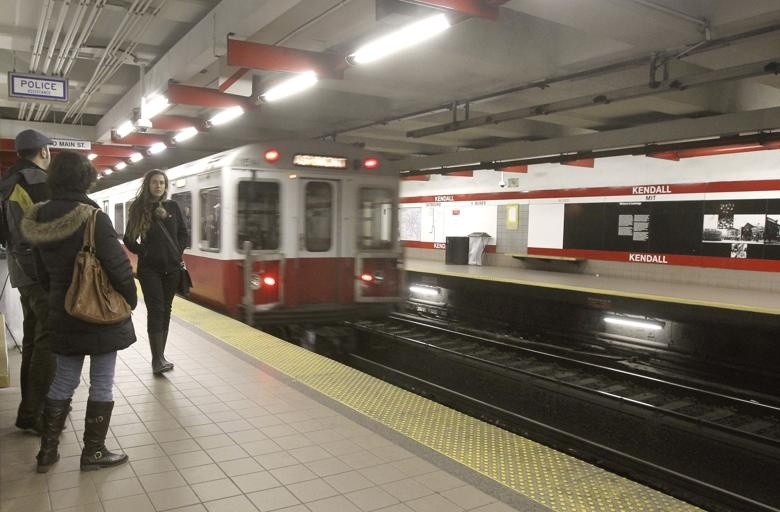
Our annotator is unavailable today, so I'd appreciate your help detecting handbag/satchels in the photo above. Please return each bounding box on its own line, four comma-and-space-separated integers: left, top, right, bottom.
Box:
64, 251, 132, 325
177, 268, 193, 298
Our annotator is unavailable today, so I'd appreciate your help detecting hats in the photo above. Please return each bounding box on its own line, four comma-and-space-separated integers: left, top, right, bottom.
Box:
15, 130, 55, 152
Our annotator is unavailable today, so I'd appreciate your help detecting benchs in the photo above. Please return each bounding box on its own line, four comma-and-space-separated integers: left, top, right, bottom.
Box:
512, 254, 585, 269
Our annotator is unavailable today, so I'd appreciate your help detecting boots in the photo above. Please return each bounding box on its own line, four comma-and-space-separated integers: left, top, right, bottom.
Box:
80, 399, 129, 471
36, 396, 72, 473
149, 330, 174, 374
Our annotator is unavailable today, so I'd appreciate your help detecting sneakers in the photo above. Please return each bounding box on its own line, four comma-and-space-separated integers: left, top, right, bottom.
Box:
16, 417, 66, 436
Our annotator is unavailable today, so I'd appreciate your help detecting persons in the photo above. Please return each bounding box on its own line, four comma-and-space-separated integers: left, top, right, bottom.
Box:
0, 130, 72, 434
20, 150, 139, 474
123, 169, 190, 373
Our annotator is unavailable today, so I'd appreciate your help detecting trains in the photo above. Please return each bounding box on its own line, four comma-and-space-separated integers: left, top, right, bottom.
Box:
87, 142, 408, 328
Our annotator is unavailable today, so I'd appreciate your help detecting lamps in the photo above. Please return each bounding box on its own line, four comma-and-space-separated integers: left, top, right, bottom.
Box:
49, 12, 474, 179
602, 312, 666, 331
409, 283, 441, 297
